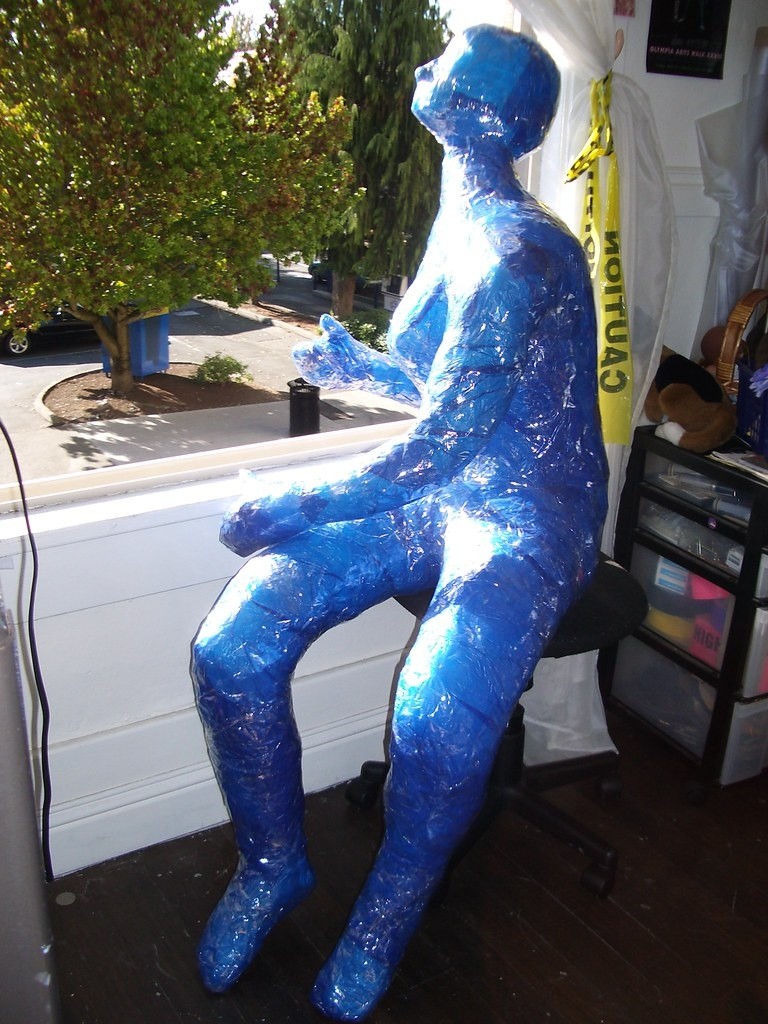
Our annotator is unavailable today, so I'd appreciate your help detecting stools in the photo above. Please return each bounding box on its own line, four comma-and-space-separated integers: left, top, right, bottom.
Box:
347, 551, 647, 893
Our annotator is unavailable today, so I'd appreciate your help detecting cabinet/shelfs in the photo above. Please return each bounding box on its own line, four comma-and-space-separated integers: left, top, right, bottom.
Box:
598, 426, 768, 805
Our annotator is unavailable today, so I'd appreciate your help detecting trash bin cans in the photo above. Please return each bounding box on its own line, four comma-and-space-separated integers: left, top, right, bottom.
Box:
286, 377, 321, 439
101, 299, 171, 383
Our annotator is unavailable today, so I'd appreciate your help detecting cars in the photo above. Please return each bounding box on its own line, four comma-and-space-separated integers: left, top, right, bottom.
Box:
308, 257, 383, 294
0, 303, 86, 357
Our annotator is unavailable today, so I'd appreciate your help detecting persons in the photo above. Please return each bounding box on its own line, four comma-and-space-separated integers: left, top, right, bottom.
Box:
187, 23, 611, 1020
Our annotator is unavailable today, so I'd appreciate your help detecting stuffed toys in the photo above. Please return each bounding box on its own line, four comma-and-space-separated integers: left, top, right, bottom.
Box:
632, 305, 748, 452
